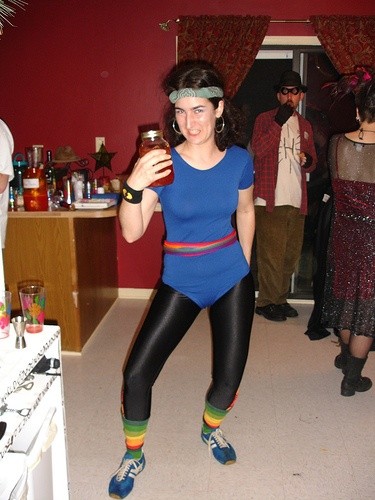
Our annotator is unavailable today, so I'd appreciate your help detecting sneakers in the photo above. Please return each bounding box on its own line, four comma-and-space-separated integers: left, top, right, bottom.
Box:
200, 428, 237, 465
108, 450, 146, 499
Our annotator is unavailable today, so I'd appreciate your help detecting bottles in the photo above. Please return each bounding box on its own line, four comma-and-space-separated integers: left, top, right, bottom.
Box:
139, 130, 174, 188
8, 145, 97, 211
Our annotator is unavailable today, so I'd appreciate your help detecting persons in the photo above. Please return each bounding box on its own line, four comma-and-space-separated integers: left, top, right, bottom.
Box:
108, 59, 257, 500
247, 70, 318, 322
0, 119, 15, 295
319, 78, 375, 397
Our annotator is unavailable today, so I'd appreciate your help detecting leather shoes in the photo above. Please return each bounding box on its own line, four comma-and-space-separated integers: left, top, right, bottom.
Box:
270, 302, 298, 318
255, 303, 287, 321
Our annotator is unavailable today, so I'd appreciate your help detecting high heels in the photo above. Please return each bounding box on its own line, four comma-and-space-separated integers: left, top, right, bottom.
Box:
341, 376, 372, 396
335, 353, 347, 375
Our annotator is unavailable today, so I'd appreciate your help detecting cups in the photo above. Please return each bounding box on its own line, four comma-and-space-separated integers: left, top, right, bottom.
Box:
0, 291, 12, 339
11, 316, 32, 345
19, 285, 45, 333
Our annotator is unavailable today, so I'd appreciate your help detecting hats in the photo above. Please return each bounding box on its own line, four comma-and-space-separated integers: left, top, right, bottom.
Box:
272, 70, 309, 92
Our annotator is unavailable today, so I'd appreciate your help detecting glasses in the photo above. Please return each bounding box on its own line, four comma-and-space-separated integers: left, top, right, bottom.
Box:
279, 87, 300, 95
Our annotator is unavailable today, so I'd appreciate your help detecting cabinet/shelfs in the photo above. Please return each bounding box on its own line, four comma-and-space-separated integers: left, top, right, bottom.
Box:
0, 323, 69, 500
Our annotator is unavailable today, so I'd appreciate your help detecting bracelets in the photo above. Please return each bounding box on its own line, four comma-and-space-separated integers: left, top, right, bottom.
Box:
121, 182, 143, 204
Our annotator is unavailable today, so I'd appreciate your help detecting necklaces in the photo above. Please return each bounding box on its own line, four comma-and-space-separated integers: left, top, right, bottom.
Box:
358, 128, 375, 140
